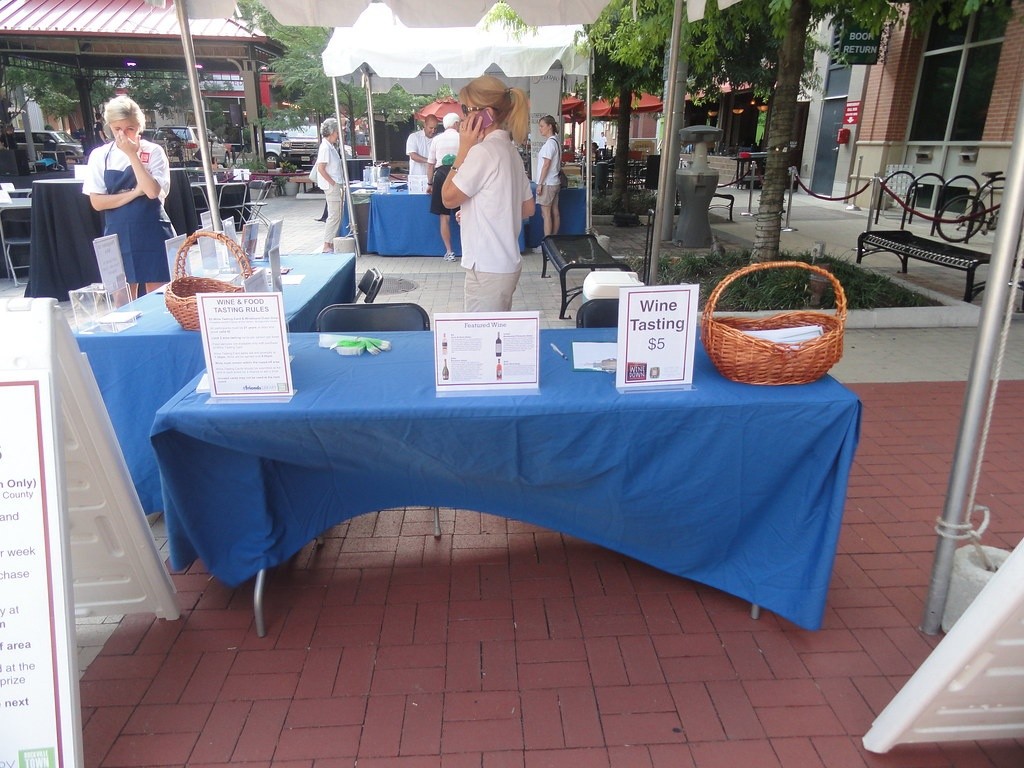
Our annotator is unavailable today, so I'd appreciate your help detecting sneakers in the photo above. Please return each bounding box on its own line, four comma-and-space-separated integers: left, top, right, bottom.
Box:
444, 253, 456, 262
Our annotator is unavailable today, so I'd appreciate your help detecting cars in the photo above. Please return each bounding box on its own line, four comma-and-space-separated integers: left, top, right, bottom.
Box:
255, 130, 284, 169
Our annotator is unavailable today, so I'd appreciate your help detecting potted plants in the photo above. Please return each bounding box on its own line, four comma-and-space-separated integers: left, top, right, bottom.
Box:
244, 156, 299, 201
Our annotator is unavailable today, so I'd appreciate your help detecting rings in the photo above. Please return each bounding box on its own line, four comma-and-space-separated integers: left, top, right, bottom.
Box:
538, 192, 539, 193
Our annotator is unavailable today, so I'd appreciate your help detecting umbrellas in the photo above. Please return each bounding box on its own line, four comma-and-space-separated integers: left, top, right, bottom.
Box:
561, 96, 585, 124
591, 91, 662, 144
415, 96, 466, 122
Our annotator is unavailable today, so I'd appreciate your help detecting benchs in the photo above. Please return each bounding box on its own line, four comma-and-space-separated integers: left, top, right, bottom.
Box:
855, 229, 993, 303
538, 231, 621, 318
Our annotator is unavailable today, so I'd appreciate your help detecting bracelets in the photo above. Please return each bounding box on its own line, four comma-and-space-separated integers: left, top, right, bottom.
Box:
538, 184, 542, 185
451, 167, 458, 171
428, 183, 432, 185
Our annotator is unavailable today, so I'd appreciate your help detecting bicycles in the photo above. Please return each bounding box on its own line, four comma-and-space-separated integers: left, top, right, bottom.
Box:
935, 170, 1006, 242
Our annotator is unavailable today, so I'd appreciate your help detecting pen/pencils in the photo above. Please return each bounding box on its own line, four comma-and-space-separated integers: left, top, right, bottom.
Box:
550, 343, 568, 360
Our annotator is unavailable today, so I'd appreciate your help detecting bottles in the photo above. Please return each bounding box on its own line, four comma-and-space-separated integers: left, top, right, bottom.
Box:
363, 166, 370, 182
377, 177, 390, 193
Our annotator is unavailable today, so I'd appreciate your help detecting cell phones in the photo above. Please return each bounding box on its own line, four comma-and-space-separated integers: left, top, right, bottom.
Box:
472, 109, 494, 132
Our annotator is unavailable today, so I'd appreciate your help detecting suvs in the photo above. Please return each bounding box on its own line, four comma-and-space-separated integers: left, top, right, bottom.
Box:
279, 125, 322, 172
141, 124, 253, 169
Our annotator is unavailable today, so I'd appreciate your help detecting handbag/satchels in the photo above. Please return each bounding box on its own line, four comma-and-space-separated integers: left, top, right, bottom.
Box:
559, 170, 568, 189
308, 160, 318, 183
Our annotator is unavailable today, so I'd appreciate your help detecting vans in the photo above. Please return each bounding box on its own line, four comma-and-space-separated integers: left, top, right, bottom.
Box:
13, 129, 85, 166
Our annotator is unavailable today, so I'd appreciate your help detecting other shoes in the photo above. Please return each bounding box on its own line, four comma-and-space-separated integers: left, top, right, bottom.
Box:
532, 245, 543, 253
314, 218, 326, 223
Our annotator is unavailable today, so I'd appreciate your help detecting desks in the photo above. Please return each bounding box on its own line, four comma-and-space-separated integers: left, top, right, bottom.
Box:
85, 252, 360, 522
150, 325, 863, 637
23, 168, 198, 301
368, 186, 527, 257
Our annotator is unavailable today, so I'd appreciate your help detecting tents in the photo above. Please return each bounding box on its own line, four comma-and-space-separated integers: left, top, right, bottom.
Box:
322, 0, 594, 260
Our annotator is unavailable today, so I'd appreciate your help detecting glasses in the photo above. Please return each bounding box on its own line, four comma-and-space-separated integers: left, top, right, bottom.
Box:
461, 104, 486, 116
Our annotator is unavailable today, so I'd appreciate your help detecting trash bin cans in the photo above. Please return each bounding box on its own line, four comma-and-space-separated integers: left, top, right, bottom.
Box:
645, 155, 660, 190
13, 148, 31, 175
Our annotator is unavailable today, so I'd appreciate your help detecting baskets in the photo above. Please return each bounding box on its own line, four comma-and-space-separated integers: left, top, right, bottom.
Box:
701, 260, 847, 386
164, 230, 254, 330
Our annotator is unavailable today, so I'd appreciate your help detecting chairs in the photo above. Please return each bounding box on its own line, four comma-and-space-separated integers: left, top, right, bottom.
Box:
350, 268, 383, 303
574, 298, 621, 327
313, 301, 431, 334
878, 165, 915, 220
1, 180, 272, 286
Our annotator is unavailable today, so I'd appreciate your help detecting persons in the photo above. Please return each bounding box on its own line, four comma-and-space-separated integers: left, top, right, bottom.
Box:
315, 202, 328, 223
405, 115, 438, 175
530, 115, 563, 254
426, 113, 461, 261
592, 142, 601, 161
599, 132, 607, 147
0, 126, 17, 149
94, 113, 107, 144
566, 137, 571, 146
316, 118, 344, 253
441, 77, 535, 312
80, 96, 177, 307
